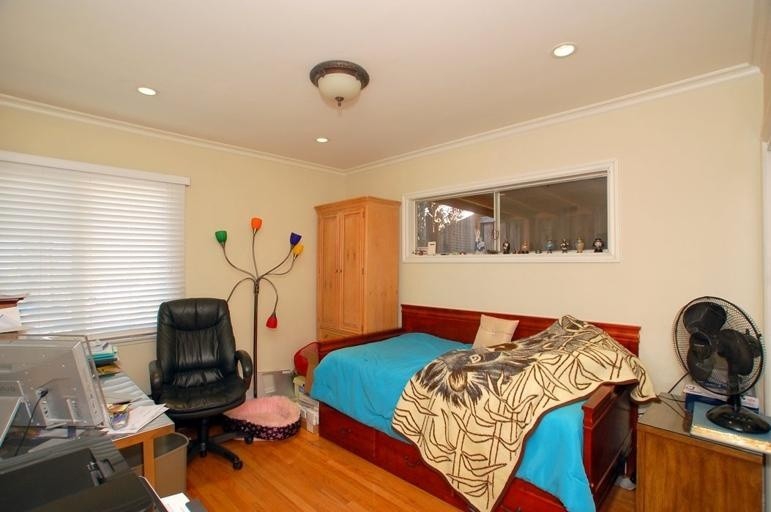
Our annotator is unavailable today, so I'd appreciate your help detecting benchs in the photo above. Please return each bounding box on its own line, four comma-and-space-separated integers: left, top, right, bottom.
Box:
314, 303, 640, 512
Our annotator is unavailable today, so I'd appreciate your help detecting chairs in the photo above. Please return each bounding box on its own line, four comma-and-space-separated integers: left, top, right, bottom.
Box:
149, 297, 254, 468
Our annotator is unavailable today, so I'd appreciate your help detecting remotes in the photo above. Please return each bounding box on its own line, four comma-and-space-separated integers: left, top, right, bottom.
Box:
112, 412, 128, 430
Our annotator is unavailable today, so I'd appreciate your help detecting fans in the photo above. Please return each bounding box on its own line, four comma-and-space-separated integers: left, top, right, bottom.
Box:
675, 297, 771, 433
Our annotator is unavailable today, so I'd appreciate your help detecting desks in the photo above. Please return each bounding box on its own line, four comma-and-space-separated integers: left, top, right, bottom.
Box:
87, 373, 174, 492
636, 395, 763, 511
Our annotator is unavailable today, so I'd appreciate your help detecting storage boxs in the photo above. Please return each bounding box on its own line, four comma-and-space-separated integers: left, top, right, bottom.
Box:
292, 375, 319, 408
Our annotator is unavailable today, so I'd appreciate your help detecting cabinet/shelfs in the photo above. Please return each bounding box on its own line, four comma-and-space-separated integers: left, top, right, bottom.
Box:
313, 196, 401, 342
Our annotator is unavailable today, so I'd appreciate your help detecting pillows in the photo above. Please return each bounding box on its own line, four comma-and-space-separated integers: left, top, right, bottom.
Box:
472, 313, 520, 350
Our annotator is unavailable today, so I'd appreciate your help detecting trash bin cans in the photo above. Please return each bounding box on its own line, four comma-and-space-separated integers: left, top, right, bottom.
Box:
120, 432, 188, 499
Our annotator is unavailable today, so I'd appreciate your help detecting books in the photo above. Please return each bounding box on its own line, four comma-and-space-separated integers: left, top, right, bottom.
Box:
0, 293, 29, 309
77, 341, 122, 377
690, 400, 771, 456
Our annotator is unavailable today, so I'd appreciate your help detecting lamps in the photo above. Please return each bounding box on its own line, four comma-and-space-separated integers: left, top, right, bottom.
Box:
215, 217, 304, 400
310, 61, 369, 108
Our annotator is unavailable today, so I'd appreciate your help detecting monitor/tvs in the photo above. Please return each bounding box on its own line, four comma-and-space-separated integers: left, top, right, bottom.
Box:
0, 336, 110, 462
